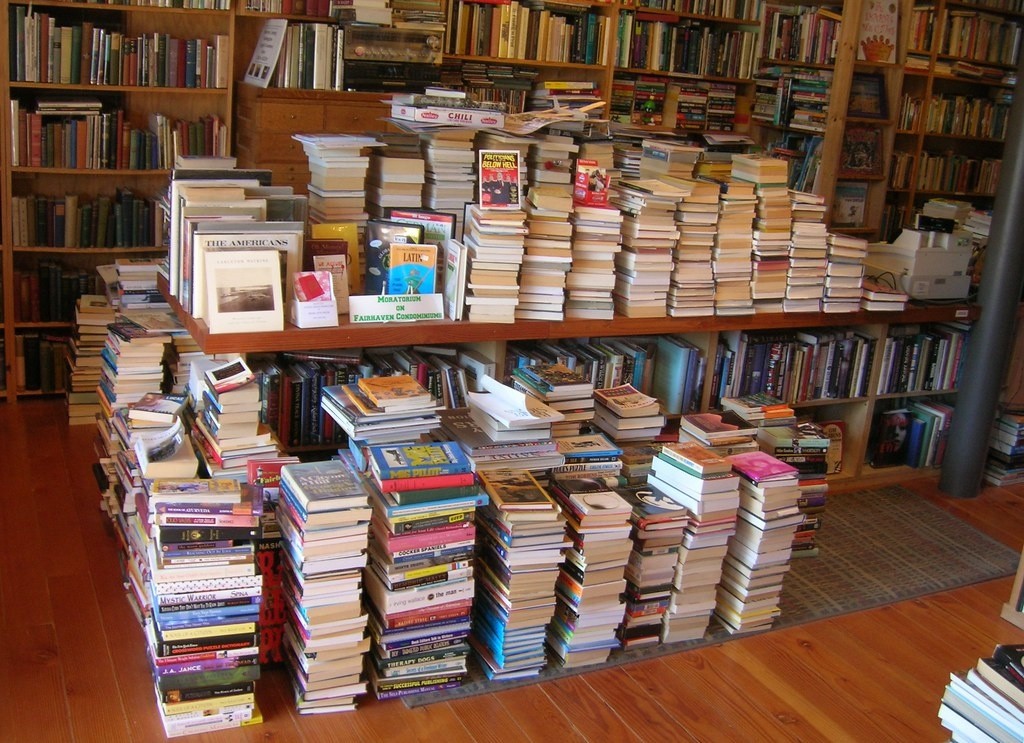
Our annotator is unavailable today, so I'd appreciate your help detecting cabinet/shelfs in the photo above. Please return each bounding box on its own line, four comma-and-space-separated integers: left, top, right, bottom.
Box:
607, 0, 766, 153
880, 0, 1024, 245
0, 0, 234, 405
156, 272, 1024, 497
441, 0, 614, 139
236, 79, 419, 220
817, 0, 911, 243
749, 0, 844, 192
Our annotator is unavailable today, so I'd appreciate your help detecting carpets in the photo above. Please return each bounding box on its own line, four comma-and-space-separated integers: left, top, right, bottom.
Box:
399, 485, 1022, 710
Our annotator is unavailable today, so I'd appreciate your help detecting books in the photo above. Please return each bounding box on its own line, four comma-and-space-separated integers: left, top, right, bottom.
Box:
1, 2, 1024, 743
